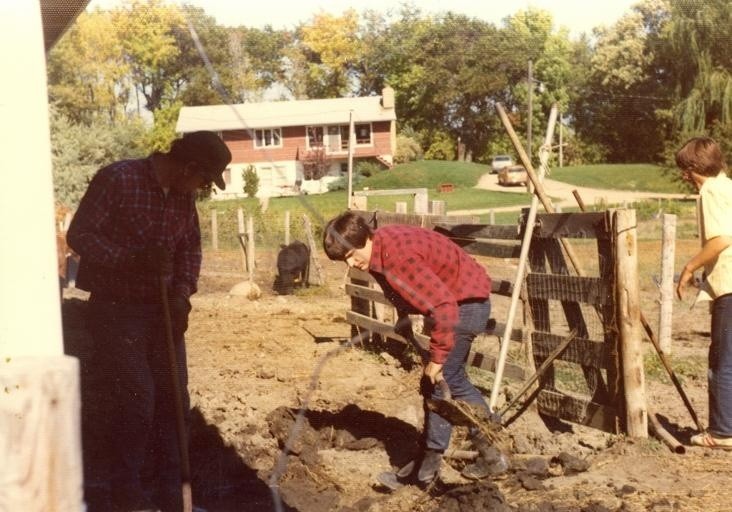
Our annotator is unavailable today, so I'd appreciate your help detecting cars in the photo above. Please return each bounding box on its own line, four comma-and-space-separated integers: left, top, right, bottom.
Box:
490, 156, 528, 186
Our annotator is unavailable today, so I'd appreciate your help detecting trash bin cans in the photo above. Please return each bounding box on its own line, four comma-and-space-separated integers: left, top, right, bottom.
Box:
441, 184, 453, 193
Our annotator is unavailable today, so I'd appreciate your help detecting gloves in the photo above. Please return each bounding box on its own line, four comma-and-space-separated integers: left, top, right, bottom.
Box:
142, 239, 179, 274
161, 289, 193, 335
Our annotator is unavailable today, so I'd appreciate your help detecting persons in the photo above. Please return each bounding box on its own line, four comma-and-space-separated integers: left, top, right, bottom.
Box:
320, 213, 510, 485
66, 130, 233, 512
672, 135, 732, 450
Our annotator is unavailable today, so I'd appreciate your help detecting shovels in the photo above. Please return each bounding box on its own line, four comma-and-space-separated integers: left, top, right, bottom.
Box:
407, 328, 482, 427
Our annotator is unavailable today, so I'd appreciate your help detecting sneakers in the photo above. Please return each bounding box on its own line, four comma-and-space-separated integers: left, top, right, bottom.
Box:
462, 454, 509, 479
418, 447, 442, 481
689, 430, 732, 449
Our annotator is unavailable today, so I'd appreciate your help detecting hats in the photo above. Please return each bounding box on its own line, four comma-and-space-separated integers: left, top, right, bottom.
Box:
181, 130, 233, 190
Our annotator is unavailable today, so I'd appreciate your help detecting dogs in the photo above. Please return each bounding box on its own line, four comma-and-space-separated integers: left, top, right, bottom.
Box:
271, 240, 311, 295
